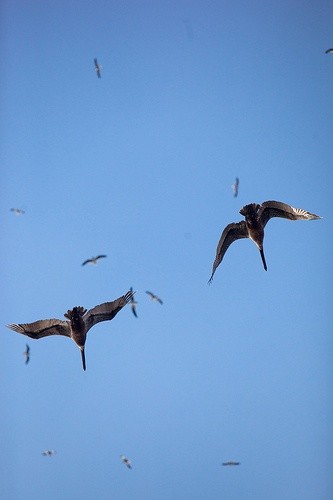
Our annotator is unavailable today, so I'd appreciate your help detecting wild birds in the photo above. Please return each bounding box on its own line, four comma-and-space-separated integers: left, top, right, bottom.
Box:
221, 460, 240, 466
4, 289, 137, 371
324, 48, 333, 54
232, 176, 240, 199
81, 254, 107, 266
93, 58, 102, 79
10, 208, 25, 215
121, 455, 132, 470
145, 291, 164, 305
23, 343, 31, 365
205, 200, 323, 287
42, 449, 56, 456
127, 286, 139, 319
